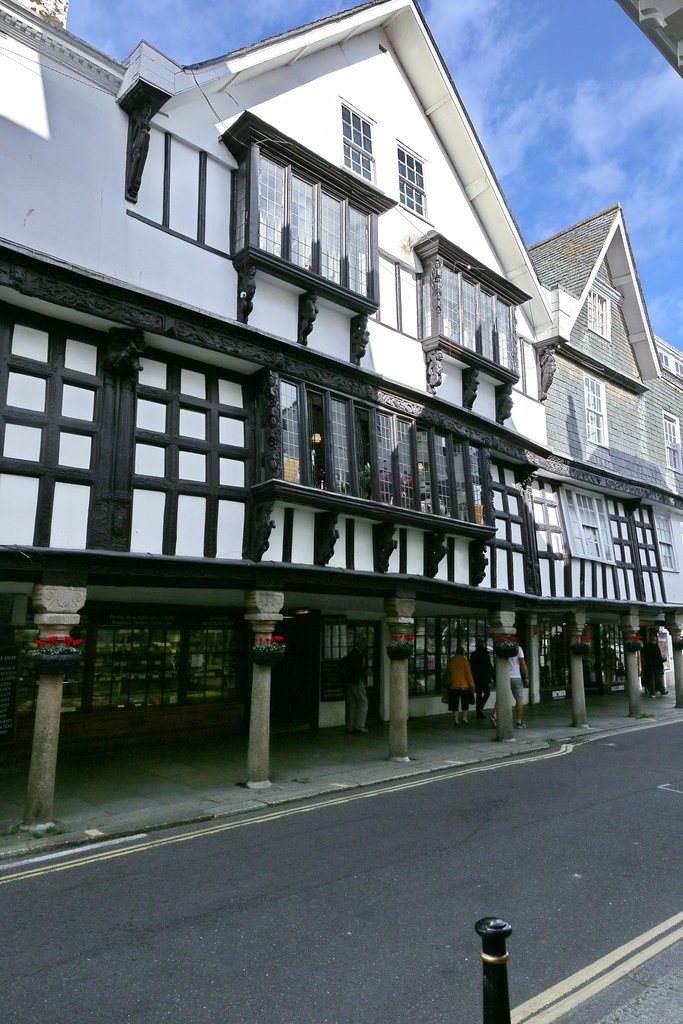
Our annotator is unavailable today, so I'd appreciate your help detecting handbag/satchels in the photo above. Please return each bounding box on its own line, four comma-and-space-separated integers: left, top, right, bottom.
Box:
441, 688, 449, 705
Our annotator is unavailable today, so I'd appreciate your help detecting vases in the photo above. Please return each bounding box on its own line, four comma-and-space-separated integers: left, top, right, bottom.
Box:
252, 651, 285, 665
496, 648, 519, 658
627, 643, 642, 652
29, 655, 81, 674
574, 649, 588, 654
387, 648, 414, 660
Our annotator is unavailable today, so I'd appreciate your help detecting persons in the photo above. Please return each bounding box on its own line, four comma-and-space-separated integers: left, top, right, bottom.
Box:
343, 638, 369, 733
490, 642, 530, 729
641, 635, 668, 697
446, 647, 476, 728
600, 639, 619, 682
470, 641, 495, 719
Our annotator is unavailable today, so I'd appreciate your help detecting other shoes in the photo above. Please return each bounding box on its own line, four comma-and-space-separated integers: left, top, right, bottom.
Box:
644, 688, 650, 694
650, 694, 656, 697
490, 716, 497, 728
476, 709, 487, 719
661, 691, 668, 695
354, 726, 368, 733
454, 722, 461, 728
516, 720, 526, 729
462, 720, 470, 726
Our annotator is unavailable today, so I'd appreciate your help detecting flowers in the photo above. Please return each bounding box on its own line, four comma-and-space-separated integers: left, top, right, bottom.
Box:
495, 636, 519, 648
386, 635, 414, 648
570, 636, 591, 650
627, 636, 642, 643
253, 635, 286, 652
32, 636, 83, 656
